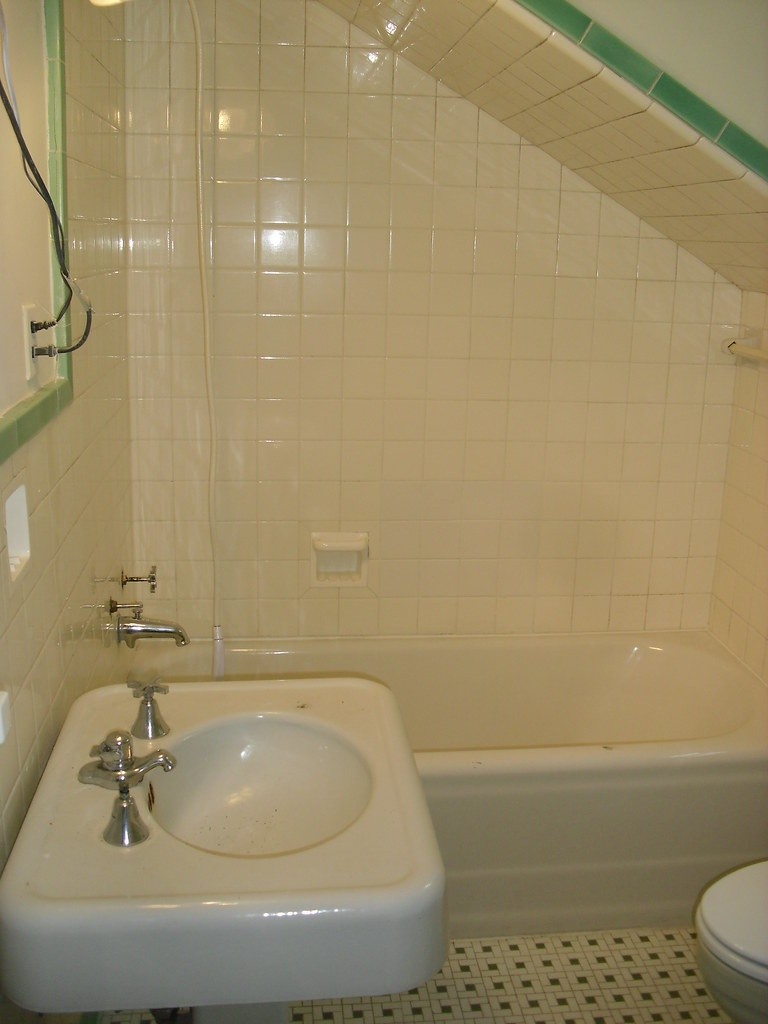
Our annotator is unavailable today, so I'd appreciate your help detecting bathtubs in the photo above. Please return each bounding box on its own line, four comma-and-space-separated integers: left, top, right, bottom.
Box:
123, 627, 767, 936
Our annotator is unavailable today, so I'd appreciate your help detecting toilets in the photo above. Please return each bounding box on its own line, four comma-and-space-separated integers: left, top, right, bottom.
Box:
692, 858, 768, 1023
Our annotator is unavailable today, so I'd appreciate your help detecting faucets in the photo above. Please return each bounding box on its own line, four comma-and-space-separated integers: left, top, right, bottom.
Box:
76, 730, 179, 791
117, 608, 192, 649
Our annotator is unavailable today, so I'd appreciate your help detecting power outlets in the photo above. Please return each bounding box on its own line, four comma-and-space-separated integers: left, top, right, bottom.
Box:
22, 302, 39, 382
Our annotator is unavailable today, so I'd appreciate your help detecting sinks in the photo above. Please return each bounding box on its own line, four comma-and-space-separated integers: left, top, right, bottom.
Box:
2, 676, 449, 1014
